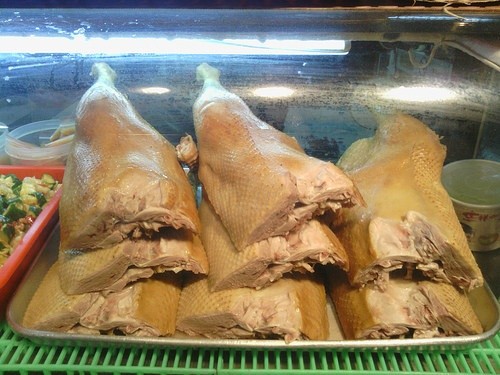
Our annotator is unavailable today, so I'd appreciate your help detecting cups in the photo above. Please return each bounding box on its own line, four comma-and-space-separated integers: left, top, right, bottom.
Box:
442, 159, 499, 251
0, 122, 10, 164
5, 119, 74, 167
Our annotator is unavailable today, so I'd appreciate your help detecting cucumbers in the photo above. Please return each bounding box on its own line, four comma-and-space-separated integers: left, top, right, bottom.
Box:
0, 171, 59, 267
